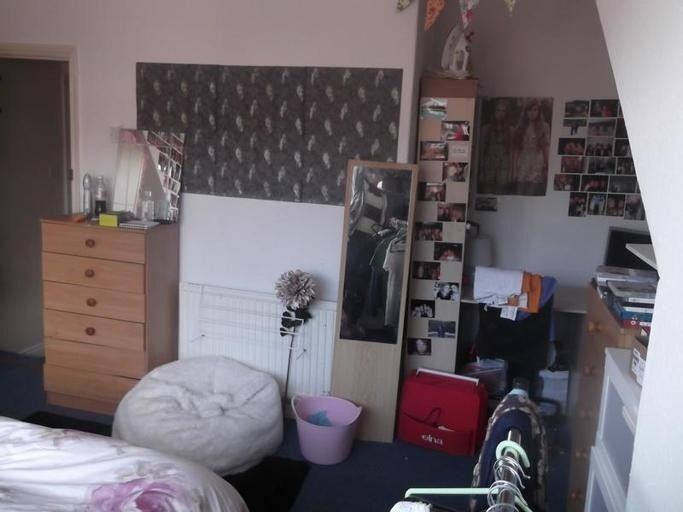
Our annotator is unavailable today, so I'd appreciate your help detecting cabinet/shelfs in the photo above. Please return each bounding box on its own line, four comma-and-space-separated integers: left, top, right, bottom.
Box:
583, 346, 642, 511
402, 76, 483, 374
567, 290, 634, 511
39, 211, 179, 426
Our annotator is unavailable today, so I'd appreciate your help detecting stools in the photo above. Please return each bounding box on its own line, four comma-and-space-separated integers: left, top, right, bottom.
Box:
111, 355, 285, 478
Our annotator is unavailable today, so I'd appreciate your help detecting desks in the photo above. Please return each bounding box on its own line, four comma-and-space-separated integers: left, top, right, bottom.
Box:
457, 275, 592, 315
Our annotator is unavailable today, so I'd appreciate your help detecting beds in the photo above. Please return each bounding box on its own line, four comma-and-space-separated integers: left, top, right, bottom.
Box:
1, 415, 249, 512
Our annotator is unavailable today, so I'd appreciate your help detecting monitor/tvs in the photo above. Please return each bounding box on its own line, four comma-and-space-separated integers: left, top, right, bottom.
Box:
604, 227, 654, 270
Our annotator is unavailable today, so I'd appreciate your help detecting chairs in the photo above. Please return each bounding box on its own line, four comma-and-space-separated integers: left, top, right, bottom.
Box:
480, 292, 564, 420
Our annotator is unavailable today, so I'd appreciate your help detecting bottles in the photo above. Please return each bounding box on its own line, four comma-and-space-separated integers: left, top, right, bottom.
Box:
82, 171, 91, 220
94, 175, 105, 219
140, 189, 153, 222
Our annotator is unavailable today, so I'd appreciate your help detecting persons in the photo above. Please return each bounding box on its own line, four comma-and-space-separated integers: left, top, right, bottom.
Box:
478, 100, 516, 193
555, 100, 645, 220
407, 94, 470, 355
512, 99, 551, 195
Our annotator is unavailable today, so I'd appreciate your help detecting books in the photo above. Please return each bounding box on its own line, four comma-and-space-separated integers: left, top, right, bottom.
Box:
594, 265, 658, 329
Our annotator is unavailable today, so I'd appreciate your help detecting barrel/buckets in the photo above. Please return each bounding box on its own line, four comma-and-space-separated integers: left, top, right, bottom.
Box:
291, 393, 361, 466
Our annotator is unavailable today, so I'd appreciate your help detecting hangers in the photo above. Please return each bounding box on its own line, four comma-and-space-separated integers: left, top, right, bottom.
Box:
403, 440, 533, 512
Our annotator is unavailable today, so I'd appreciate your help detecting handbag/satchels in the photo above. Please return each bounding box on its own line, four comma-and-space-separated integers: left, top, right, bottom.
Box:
396, 367, 488, 457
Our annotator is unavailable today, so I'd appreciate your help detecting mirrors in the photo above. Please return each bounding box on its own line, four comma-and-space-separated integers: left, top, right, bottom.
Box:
331, 158, 418, 446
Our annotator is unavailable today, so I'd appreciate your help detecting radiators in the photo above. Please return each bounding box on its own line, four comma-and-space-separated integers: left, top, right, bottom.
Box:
178, 281, 337, 399
